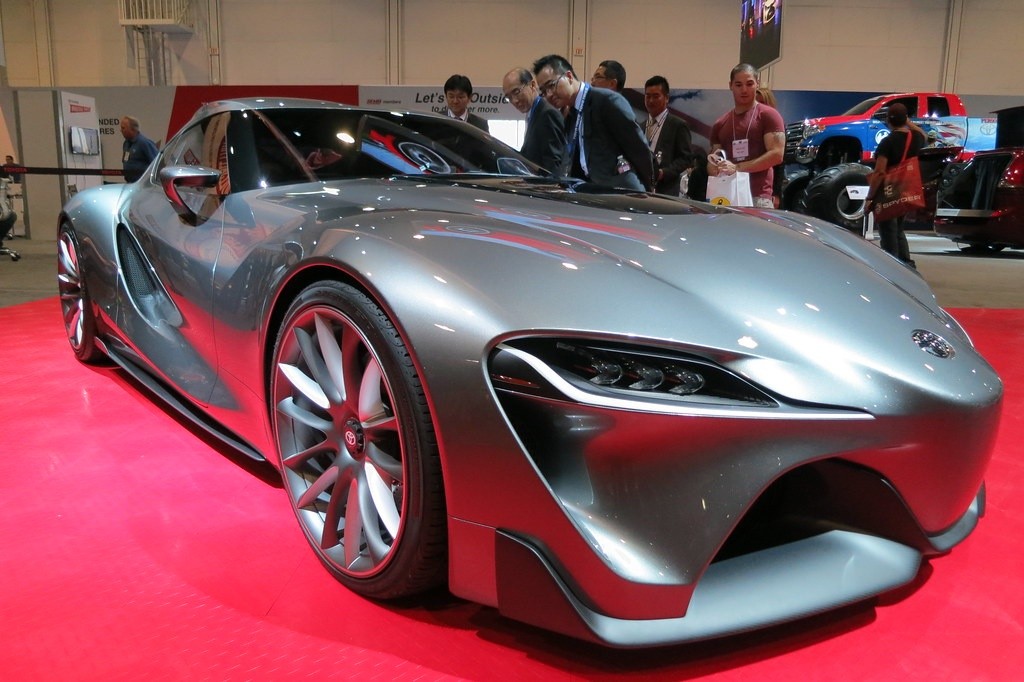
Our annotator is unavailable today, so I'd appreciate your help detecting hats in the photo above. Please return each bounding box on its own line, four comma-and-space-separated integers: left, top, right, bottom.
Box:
928, 130, 937, 138
888, 103, 907, 127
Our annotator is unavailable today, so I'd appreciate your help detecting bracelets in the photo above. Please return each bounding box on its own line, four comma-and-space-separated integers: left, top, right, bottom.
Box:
866, 198, 874, 202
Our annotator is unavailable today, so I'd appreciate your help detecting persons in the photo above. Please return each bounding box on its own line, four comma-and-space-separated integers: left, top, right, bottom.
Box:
0, 155, 22, 183
503, 68, 568, 176
120, 116, 159, 184
755, 88, 785, 209
864, 103, 929, 270
438, 75, 490, 134
590, 59, 626, 94
707, 63, 786, 210
687, 144, 707, 202
640, 76, 693, 197
533, 55, 660, 192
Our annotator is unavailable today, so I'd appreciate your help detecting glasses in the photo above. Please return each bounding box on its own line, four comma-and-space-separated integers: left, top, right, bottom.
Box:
590, 76, 610, 81
503, 82, 528, 104
539, 73, 565, 97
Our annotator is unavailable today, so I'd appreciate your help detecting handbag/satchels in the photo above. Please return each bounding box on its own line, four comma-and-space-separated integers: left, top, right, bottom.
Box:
866, 130, 926, 223
704, 149, 753, 207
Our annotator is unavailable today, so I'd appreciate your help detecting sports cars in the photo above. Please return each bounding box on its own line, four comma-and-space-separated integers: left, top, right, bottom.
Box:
56, 96, 1004, 649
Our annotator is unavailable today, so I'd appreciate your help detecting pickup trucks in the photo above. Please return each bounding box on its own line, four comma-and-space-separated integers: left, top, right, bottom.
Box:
783, 91, 998, 233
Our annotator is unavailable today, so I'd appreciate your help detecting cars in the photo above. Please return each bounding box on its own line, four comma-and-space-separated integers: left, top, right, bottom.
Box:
932, 104, 1024, 253
